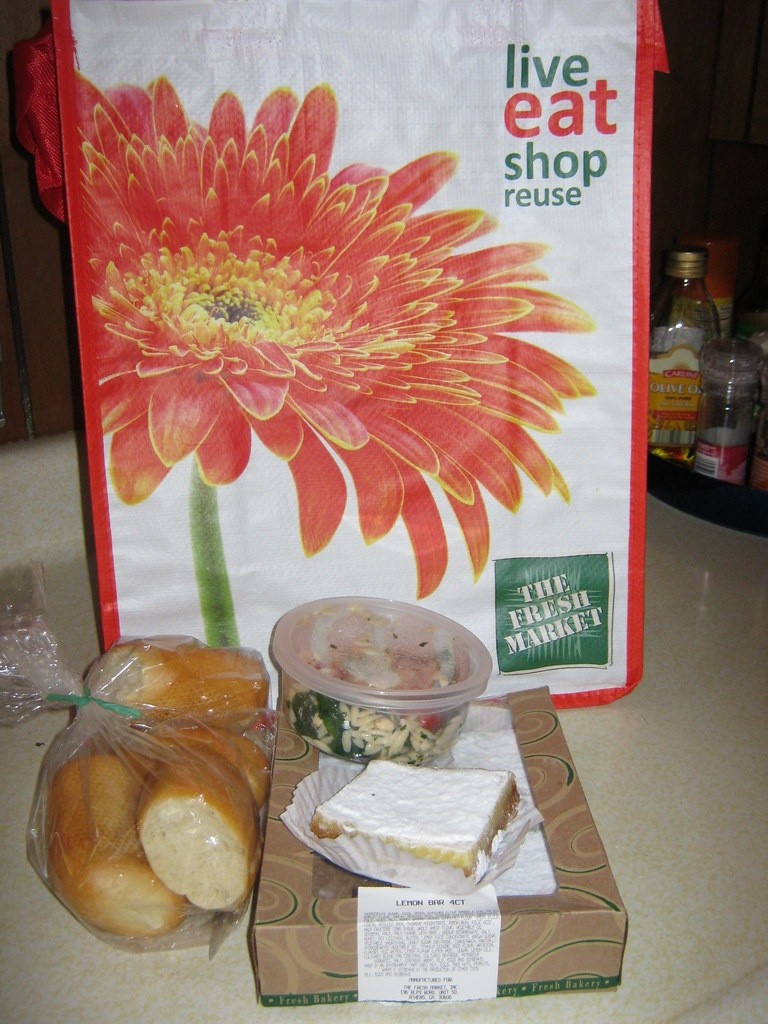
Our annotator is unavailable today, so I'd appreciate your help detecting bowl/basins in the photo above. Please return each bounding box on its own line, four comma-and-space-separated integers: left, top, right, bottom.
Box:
269, 594, 493, 768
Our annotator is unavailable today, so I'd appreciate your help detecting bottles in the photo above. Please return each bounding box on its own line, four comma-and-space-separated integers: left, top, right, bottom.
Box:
751, 367, 768, 496
648, 247, 723, 466
732, 213, 768, 338
691, 337, 764, 483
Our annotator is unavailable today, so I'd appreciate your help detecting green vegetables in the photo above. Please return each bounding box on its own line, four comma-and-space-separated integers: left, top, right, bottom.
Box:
288, 690, 429, 767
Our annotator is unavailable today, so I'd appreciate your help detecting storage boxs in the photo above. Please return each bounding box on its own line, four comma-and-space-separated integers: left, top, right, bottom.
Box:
239, 682, 630, 1011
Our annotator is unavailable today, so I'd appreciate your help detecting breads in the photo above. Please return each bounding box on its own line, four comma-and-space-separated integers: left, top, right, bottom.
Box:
309, 760, 520, 877
45, 637, 272, 940
